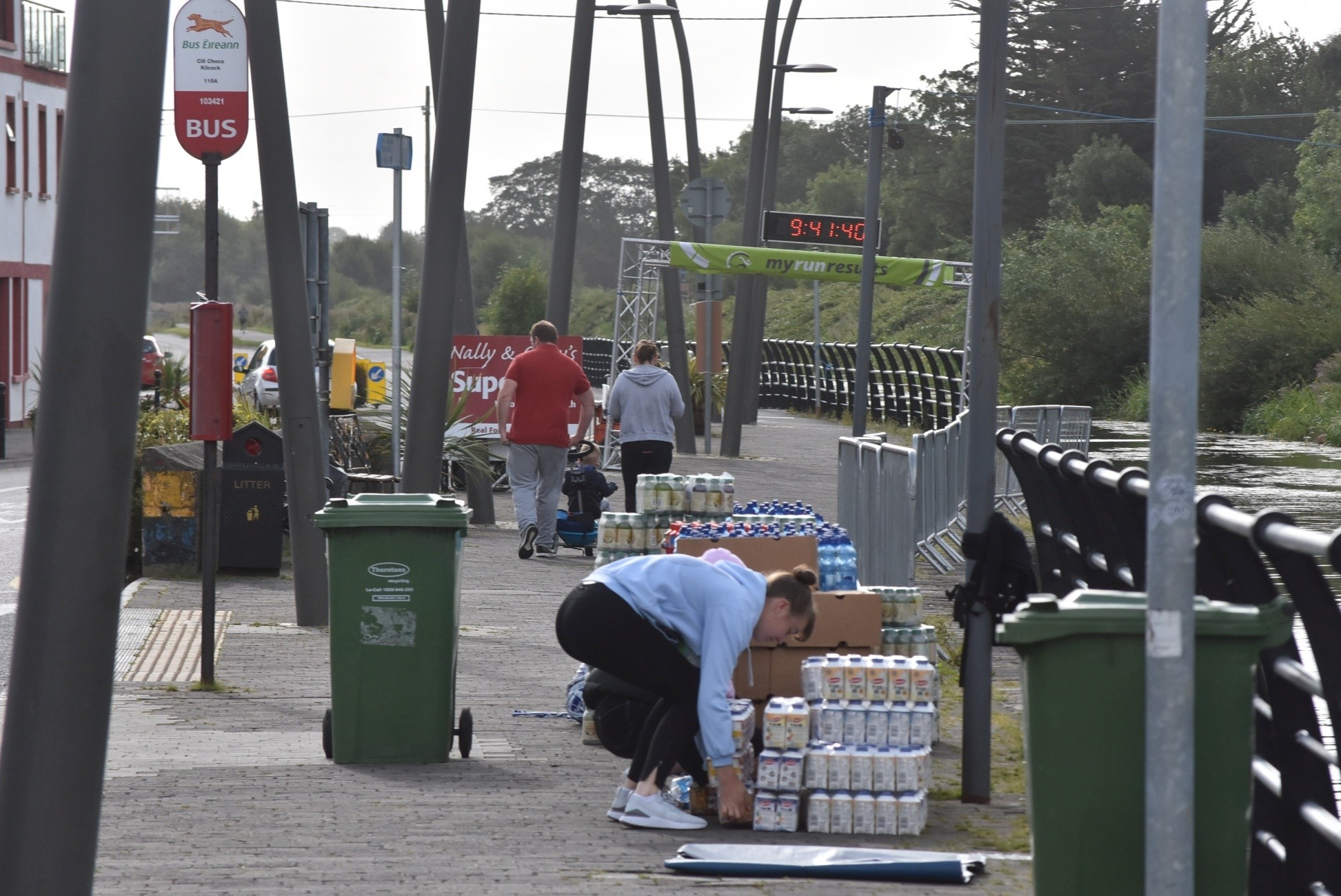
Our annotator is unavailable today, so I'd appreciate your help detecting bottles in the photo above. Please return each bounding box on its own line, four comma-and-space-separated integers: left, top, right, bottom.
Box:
592, 473, 935, 836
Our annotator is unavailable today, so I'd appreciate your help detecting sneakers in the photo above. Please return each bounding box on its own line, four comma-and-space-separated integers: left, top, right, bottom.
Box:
518, 525, 539, 559
606, 785, 708, 830
535, 543, 557, 557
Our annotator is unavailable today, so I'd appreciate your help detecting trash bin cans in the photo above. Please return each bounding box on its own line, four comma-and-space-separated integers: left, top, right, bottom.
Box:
312, 494, 474, 767
995, 586, 1259, 896
218, 421, 286, 578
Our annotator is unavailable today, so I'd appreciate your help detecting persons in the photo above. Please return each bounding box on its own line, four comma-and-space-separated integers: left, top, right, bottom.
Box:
560, 440, 620, 523
607, 338, 686, 514
554, 546, 819, 831
495, 319, 595, 560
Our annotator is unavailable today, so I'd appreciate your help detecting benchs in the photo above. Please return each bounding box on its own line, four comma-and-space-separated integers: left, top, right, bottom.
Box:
329, 413, 399, 494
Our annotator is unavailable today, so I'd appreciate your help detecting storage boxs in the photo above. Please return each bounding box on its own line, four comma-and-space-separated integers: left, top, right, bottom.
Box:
676, 535, 882, 750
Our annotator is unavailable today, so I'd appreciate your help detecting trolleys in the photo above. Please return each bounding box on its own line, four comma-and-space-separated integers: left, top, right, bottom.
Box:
555, 441, 608, 549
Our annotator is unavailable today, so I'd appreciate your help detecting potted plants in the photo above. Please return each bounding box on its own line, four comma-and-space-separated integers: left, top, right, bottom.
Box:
27, 346, 42, 444
659, 356, 729, 436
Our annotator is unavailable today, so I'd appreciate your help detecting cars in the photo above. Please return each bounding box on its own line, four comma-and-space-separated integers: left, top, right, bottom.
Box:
231, 332, 356, 414
